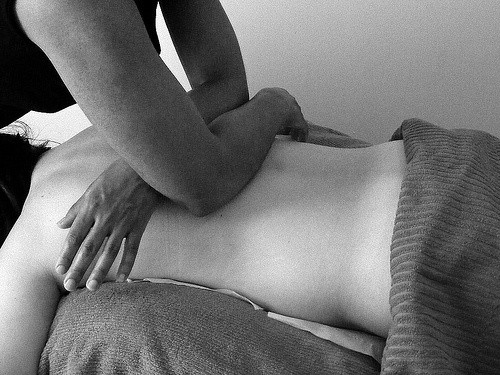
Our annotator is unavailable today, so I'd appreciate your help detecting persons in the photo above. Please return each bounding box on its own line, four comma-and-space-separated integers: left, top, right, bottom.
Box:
1, 119, 498, 372
1, 0, 307, 294
